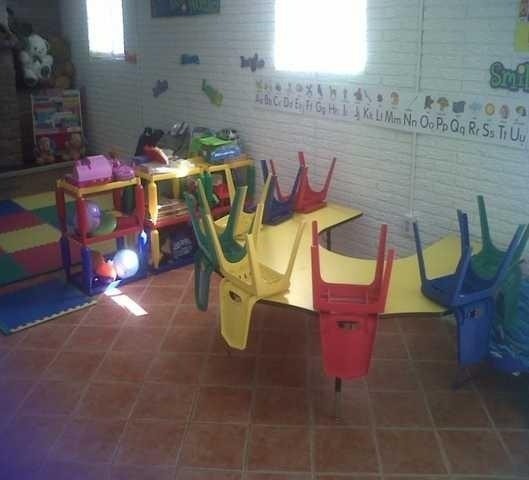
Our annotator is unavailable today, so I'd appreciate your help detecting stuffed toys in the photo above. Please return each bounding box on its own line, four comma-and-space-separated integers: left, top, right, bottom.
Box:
19, 33, 74, 90
33, 137, 55, 165
64, 134, 88, 160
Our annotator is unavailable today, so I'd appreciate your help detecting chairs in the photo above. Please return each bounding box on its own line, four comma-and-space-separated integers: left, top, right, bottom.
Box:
457, 195, 529, 330
205, 204, 306, 348
182, 152, 337, 309
311, 220, 395, 380
414, 213, 527, 368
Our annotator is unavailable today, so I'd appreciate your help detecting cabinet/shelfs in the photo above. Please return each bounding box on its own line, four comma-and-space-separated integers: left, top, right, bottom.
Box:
53, 146, 257, 302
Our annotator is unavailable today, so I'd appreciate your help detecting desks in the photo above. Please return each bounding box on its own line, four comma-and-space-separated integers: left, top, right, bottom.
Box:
211, 203, 483, 420
